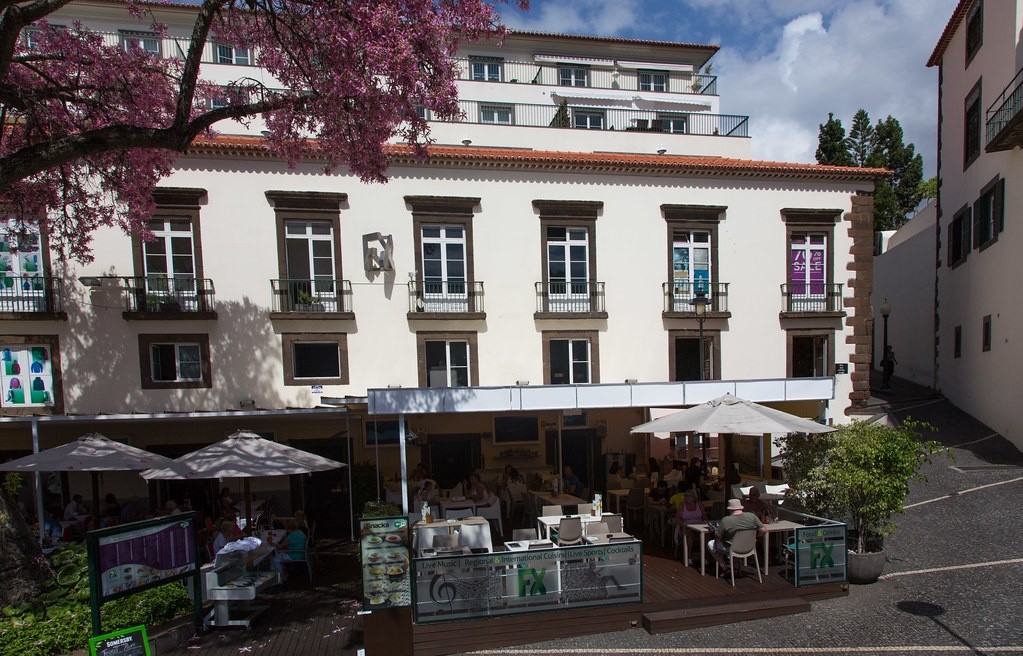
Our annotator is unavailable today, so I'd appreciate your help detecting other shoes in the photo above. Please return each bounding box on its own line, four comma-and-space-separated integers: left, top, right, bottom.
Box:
688, 559, 693, 566
770, 557, 780, 566
735, 568, 741, 578
720, 568, 731, 578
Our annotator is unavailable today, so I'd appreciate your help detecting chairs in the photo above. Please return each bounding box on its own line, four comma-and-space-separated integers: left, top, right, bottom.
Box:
57, 499, 318, 581
409, 472, 796, 587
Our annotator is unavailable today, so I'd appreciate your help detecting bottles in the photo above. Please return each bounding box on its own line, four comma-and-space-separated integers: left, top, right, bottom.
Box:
760, 515, 769, 524
255, 525, 265, 540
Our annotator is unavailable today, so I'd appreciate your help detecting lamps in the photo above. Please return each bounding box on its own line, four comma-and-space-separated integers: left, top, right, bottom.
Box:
657, 150, 666, 155
612, 67, 620, 78
462, 140, 471, 146
261, 130, 271, 135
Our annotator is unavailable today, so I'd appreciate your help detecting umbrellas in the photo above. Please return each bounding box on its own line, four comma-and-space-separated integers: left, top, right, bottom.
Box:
0, 432, 174, 530
629, 393, 839, 516
139, 428, 348, 537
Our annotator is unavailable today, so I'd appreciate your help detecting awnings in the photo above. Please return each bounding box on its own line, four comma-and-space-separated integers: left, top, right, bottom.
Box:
367, 376, 835, 516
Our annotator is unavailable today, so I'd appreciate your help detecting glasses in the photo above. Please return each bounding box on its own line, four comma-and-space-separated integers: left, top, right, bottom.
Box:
749, 493, 759, 496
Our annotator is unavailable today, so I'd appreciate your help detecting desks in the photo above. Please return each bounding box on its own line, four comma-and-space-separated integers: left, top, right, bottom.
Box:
413, 516, 493, 575
583, 532, 639, 562
607, 490, 627, 513
648, 501, 718, 548
225, 526, 284, 570
681, 520, 805, 575
539, 493, 587, 506
538, 512, 623, 540
438, 497, 475, 519
504, 539, 561, 603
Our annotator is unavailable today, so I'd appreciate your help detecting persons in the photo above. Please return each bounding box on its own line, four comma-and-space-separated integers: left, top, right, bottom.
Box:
185, 479, 211, 513
561, 465, 580, 497
609, 455, 741, 550
498, 464, 528, 501
12, 494, 61, 539
885, 345, 898, 383
741, 487, 783, 565
409, 463, 439, 506
707, 499, 766, 577
676, 489, 708, 566
212, 487, 309, 586
98, 493, 121, 528
64, 495, 88, 521
463, 472, 489, 503
121, 495, 193, 545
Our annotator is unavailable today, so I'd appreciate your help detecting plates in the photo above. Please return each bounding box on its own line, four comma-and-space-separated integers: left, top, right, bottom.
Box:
367, 535, 403, 605
452, 497, 465, 501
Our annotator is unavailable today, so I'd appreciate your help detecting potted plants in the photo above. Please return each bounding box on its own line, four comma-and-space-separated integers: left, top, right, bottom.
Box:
295, 290, 325, 311
773, 415, 957, 583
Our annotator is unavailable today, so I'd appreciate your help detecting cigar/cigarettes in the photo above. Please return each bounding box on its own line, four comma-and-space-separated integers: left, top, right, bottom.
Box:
464, 478, 465, 481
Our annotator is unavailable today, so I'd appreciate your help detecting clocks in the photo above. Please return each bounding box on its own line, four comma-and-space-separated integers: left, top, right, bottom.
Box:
597, 424, 608, 436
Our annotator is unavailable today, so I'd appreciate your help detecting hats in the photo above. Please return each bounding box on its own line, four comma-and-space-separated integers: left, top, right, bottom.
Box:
727, 499, 744, 510
